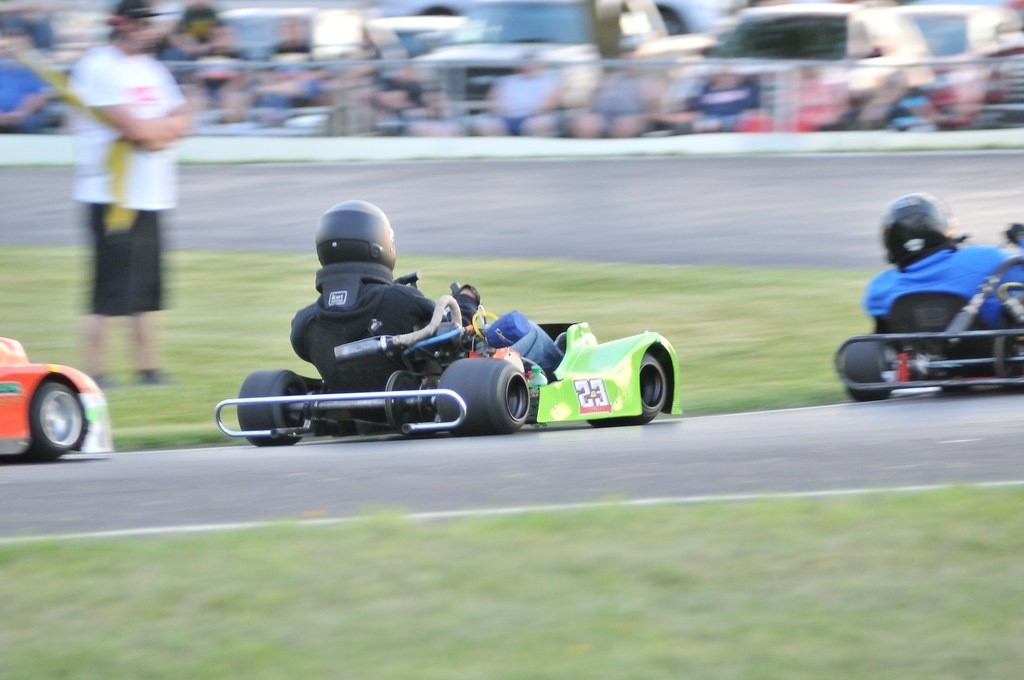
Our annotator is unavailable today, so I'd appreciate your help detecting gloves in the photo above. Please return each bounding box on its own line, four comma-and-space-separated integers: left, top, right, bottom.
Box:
1006, 222, 1024, 243
450, 281, 481, 304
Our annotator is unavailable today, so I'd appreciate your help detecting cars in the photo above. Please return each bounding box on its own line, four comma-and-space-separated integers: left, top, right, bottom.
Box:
0, 0, 1023, 140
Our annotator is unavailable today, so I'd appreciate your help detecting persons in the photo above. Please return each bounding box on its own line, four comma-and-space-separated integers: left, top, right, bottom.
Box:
0, 27, 69, 133
118, 0, 252, 122
863, 193, 1024, 358
67, 0, 194, 383
290, 198, 564, 392
264, 18, 986, 138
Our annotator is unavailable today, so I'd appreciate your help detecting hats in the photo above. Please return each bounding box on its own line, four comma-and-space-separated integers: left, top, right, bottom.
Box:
116, 0, 160, 19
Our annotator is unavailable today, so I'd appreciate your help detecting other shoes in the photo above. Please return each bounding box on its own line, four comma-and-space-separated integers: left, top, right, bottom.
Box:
95, 374, 115, 390
135, 367, 168, 383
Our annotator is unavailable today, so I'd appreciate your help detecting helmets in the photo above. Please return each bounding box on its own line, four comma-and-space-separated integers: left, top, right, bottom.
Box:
881, 195, 950, 259
317, 200, 396, 271
